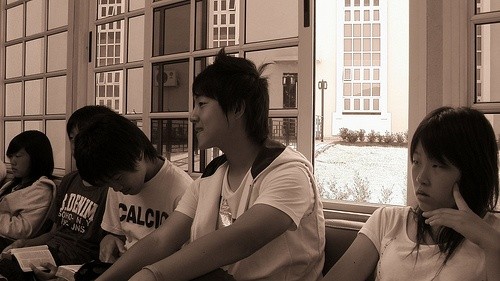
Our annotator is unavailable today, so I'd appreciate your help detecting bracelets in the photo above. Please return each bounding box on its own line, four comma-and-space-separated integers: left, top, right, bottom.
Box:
143, 264, 163, 281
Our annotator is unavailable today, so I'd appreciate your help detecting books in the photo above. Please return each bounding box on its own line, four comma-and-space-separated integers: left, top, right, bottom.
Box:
3, 245, 57, 272
55, 264, 84, 281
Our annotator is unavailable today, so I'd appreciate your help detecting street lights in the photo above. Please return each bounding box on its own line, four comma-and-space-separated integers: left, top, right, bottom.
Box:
318, 79, 328, 142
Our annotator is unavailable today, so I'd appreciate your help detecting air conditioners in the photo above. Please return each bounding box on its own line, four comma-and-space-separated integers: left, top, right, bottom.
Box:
154, 70, 180, 87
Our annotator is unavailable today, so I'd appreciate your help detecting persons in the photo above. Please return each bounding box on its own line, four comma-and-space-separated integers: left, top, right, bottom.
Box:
95, 46, 325, 281
0, 105, 112, 281
322, 106, 500, 281
0, 131, 56, 253
73, 111, 194, 281
